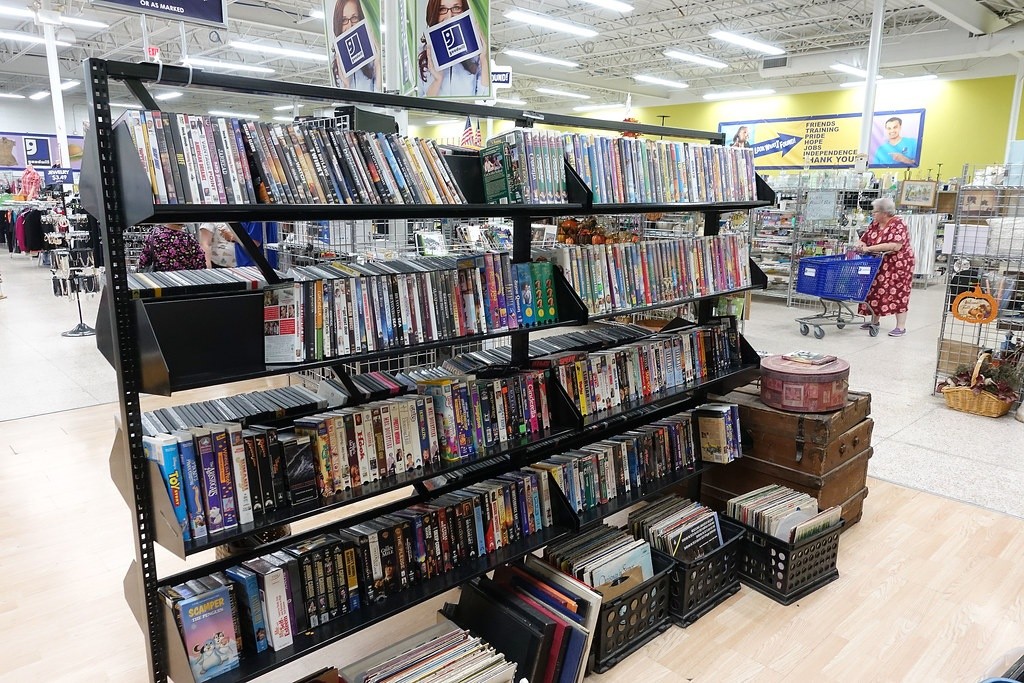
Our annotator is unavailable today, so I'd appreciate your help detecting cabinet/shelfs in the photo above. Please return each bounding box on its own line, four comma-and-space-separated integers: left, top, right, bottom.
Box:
752, 173, 885, 313
71, 54, 777, 683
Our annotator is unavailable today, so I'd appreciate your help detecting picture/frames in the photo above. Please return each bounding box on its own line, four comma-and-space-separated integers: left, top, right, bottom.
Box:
718, 108, 926, 168
962, 189, 996, 212
899, 180, 937, 207
951, 285, 998, 323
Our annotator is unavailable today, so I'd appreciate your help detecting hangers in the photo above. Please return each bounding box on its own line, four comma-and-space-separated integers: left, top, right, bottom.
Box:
1, 204, 60, 215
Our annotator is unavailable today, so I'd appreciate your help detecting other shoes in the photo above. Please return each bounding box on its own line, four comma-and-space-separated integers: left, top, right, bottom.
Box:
888, 328, 906, 336
859, 322, 880, 330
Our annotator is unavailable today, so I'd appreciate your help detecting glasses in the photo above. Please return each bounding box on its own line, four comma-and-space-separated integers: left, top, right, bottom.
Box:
871, 210, 881, 214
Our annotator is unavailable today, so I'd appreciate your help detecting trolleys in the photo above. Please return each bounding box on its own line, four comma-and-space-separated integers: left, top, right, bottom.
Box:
795, 250, 894, 340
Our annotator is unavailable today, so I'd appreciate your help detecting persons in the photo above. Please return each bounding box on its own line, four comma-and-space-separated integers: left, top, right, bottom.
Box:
219, 222, 279, 269
350, 465, 361, 487
855, 197, 915, 337
432, 441, 438, 462
0, 137, 18, 166
406, 454, 414, 469
204, 188, 210, 196
197, 118, 203, 134
135, 224, 206, 273
730, 126, 750, 148
492, 156, 502, 168
323, 284, 328, 301
396, 449, 403, 462
388, 453, 395, 469
485, 158, 494, 173
330, 0, 381, 93
874, 117, 917, 164
200, 222, 238, 269
22, 165, 40, 201
417, 0, 490, 99
521, 284, 531, 304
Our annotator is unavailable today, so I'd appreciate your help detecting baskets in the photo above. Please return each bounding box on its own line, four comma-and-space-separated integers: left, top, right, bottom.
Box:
941, 353, 1013, 417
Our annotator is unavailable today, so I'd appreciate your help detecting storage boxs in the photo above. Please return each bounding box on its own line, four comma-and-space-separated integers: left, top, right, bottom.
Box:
700, 380, 876, 538
758, 353, 851, 413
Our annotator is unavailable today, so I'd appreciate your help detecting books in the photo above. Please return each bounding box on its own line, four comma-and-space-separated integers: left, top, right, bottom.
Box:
110, 110, 842, 683
